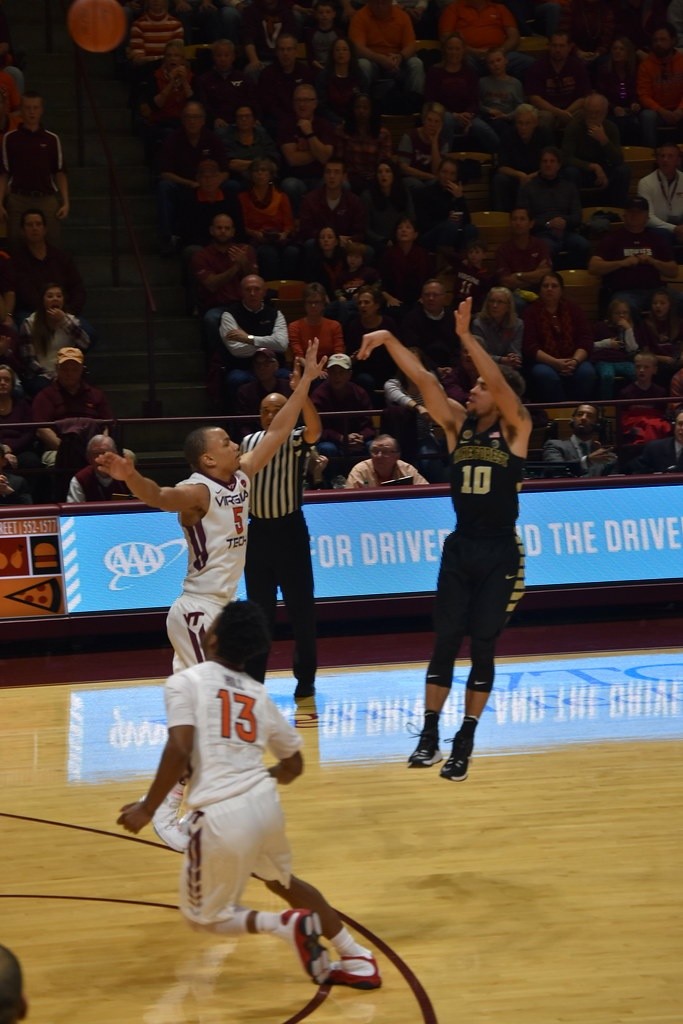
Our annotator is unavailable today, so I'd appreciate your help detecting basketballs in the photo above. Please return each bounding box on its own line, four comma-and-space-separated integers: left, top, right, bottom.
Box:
67, 0, 129, 51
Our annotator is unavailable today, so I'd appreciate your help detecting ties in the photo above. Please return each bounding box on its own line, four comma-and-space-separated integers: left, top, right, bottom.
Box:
580, 442, 588, 457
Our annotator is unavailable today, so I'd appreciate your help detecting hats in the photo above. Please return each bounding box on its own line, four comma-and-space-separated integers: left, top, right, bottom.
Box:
328, 353, 352, 370
56, 346, 84, 364
625, 197, 648, 210
252, 346, 276, 358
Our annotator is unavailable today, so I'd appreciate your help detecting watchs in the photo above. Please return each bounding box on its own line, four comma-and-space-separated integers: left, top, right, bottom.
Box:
247, 335, 254, 344
516, 271, 522, 279
546, 220, 550, 227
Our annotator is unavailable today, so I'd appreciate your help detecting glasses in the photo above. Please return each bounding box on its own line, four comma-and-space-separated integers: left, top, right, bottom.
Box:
369, 446, 400, 456
253, 360, 276, 369
293, 96, 315, 105
486, 297, 510, 306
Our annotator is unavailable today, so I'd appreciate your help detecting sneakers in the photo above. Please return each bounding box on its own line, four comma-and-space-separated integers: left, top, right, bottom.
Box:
406, 722, 442, 767
328, 953, 381, 990
440, 737, 470, 781
139, 795, 191, 851
279, 910, 329, 984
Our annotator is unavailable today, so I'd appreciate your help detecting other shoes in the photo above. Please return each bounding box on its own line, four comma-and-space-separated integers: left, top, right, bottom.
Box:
294, 680, 315, 697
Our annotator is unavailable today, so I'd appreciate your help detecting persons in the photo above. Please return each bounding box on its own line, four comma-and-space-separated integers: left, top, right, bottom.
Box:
0, 944, 28, 1024
0, 7, 134, 504
117, 601, 383, 991
237, 357, 323, 727
356, 297, 533, 782
95, 337, 327, 853
121, 0, 683, 489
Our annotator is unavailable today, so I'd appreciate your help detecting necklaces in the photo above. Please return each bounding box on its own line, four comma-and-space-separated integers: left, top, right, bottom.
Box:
0, 407, 8, 412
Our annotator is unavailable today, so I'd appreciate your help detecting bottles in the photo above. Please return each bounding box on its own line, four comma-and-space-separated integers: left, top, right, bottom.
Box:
513, 288, 538, 301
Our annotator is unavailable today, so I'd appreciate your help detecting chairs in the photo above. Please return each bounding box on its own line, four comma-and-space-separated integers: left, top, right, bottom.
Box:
0, 0, 683, 504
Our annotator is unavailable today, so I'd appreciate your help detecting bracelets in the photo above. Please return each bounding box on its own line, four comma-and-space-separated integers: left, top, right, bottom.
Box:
7, 312, 13, 316
587, 457, 593, 465
343, 433, 348, 444
304, 132, 316, 139
573, 358, 580, 366
